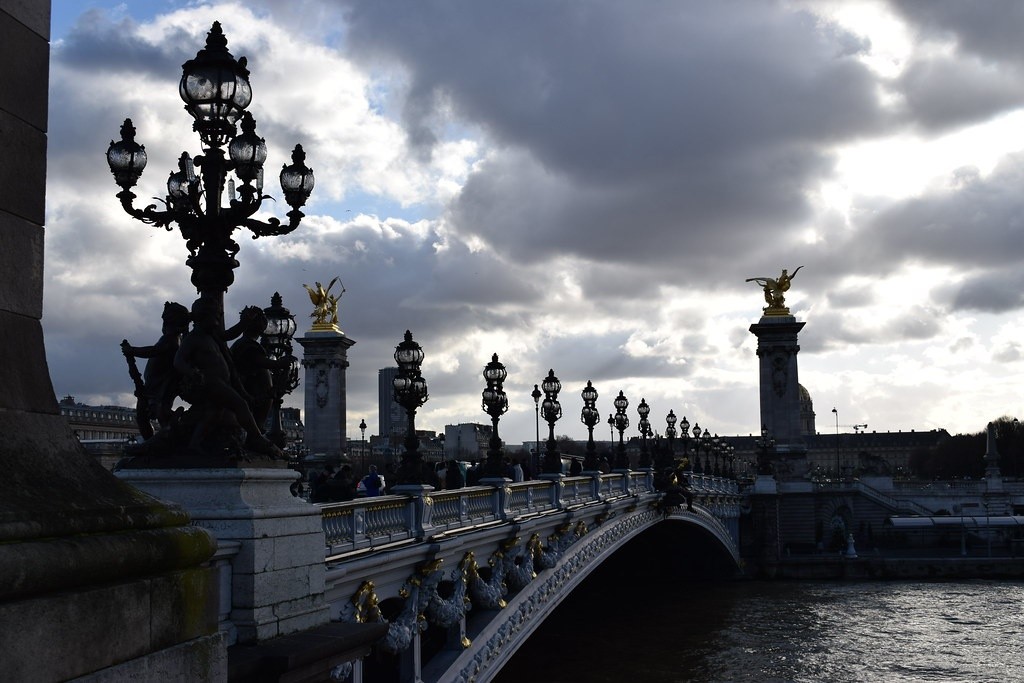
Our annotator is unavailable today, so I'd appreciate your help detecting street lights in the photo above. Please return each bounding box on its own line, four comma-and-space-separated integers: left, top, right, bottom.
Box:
712, 434, 721, 476
440, 433, 445, 461
389, 330, 431, 484
106, 22, 314, 353
680, 417, 692, 471
260, 292, 301, 451
581, 380, 601, 471
608, 414, 615, 447
531, 385, 542, 476
755, 423, 776, 475
540, 368, 562, 474
666, 410, 677, 468
612, 390, 631, 469
693, 423, 703, 473
690, 443, 696, 470
703, 429, 713, 475
482, 352, 513, 476
832, 409, 840, 478
360, 419, 367, 477
720, 436, 757, 477
638, 399, 652, 468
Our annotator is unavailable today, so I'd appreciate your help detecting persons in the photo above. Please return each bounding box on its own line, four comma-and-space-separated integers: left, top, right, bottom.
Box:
308, 463, 398, 502
120, 296, 287, 455
465, 457, 524, 487
569, 458, 582, 477
425, 460, 464, 491
